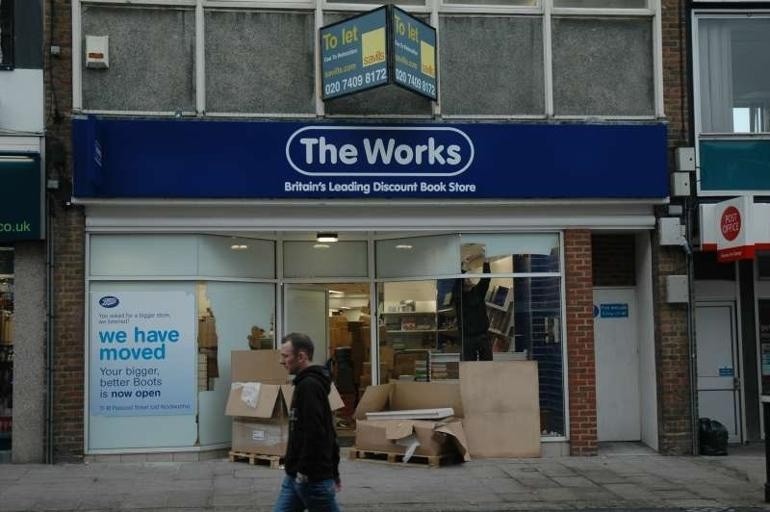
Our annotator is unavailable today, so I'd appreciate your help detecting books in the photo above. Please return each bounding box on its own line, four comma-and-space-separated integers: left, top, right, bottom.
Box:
385, 304, 449, 380
487, 287, 510, 351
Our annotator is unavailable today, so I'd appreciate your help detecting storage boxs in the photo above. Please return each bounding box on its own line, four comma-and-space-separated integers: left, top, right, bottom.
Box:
225, 348, 346, 455
352, 379, 473, 463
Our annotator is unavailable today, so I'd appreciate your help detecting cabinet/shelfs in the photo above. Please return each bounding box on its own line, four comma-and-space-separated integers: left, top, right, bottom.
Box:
380, 278, 465, 361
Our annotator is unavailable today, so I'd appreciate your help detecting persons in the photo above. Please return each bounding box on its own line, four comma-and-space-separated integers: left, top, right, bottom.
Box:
270, 332, 342, 511
450, 261, 494, 362
